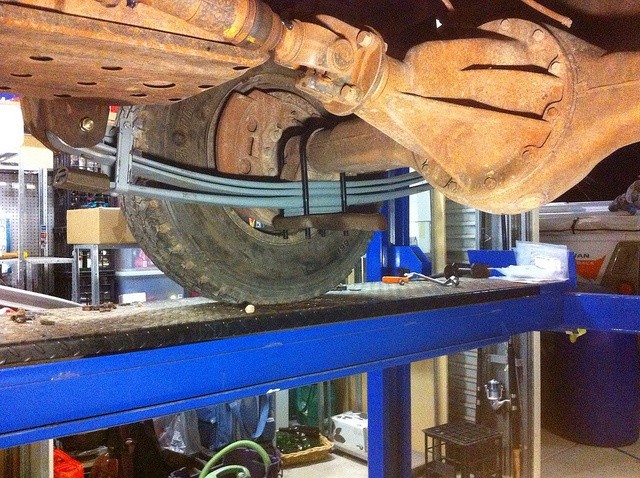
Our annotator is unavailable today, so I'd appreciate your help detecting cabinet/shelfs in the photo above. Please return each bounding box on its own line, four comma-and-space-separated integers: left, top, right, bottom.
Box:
424, 421, 504, 477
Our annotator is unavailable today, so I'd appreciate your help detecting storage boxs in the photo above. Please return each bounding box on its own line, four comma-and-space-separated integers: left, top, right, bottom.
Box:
65, 206, 137, 245
328, 412, 369, 457
